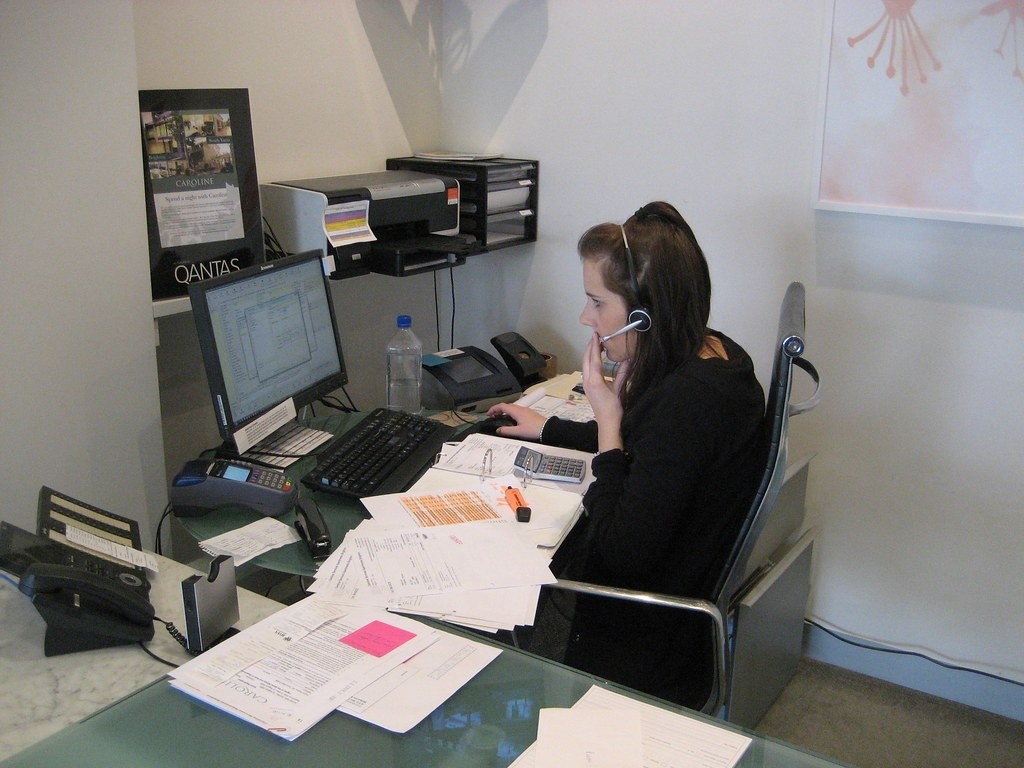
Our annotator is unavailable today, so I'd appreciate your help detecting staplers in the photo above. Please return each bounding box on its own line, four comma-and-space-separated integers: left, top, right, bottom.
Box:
294, 498, 330, 560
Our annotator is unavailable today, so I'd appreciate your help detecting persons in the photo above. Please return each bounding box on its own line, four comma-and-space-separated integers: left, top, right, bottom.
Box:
486, 201, 768, 711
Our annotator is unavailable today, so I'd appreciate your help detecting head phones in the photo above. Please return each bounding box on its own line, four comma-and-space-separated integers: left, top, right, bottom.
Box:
619, 223, 652, 331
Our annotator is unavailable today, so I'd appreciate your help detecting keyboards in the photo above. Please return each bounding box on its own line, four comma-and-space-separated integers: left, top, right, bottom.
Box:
300, 408, 457, 504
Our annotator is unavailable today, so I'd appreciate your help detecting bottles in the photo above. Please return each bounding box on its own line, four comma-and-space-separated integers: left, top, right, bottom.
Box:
386, 315, 423, 415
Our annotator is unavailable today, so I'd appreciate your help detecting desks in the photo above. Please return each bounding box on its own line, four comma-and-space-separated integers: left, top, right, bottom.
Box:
0, 551, 853, 768
178, 410, 489, 586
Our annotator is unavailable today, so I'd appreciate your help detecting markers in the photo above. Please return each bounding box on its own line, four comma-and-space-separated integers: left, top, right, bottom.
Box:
506, 486, 531, 522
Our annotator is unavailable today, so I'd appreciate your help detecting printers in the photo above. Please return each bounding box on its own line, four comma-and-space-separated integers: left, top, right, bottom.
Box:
259, 169, 469, 281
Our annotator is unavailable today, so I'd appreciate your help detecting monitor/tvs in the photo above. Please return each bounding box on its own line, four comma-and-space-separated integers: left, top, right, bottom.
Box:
187, 248, 349, 451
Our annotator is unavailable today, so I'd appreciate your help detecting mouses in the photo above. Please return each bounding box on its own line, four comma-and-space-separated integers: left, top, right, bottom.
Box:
479, 414, 518, 435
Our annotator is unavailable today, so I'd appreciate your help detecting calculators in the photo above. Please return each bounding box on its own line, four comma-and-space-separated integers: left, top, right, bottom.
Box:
513, 446, 586, 483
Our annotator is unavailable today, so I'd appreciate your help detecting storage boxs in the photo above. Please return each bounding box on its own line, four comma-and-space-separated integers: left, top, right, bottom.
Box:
388, 154, 540, 250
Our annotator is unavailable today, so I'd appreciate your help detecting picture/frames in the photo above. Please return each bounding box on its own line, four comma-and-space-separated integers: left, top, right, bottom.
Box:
138, 88, 267, 302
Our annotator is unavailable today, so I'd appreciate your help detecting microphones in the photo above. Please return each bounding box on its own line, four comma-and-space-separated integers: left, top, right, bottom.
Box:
599, 320, 641, 343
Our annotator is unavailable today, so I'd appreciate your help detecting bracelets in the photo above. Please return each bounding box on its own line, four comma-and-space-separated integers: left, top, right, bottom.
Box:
539, 418, 550, 442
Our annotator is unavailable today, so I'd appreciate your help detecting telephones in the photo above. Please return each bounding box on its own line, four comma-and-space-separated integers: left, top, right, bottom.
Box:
0, 521, 155, 656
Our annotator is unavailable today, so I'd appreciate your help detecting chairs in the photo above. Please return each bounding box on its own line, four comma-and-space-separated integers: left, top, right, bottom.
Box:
546, 279, 809, 717
